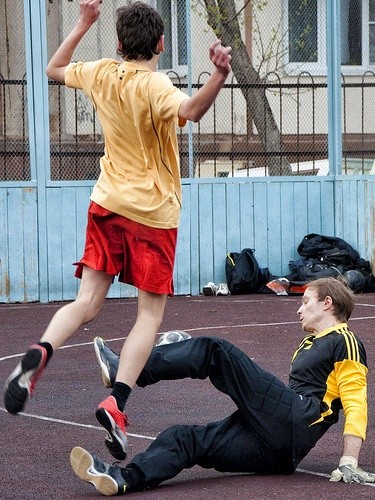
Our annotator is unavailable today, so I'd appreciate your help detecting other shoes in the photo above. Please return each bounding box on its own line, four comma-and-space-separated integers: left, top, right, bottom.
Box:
203, 282, 231, 296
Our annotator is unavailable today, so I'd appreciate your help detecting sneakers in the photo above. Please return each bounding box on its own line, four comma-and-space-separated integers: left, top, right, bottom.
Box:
94, 336, 120, 388
95, 395, 130, 460
3, 342, 48, 416
69, 446, 133, 496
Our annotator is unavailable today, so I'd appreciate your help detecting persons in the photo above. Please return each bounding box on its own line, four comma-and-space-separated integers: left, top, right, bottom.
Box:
4, 0, 231, 460
70, 277, 375, 495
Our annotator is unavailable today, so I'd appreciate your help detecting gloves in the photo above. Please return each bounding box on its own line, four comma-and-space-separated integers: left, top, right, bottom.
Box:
329, 456, 375, 485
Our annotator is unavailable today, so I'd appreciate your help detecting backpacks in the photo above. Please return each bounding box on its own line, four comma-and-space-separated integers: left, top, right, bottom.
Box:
225, 248, 262, 294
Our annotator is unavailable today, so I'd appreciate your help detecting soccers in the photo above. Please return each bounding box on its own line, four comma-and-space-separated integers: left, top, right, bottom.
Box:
156, 328, 194, 347
